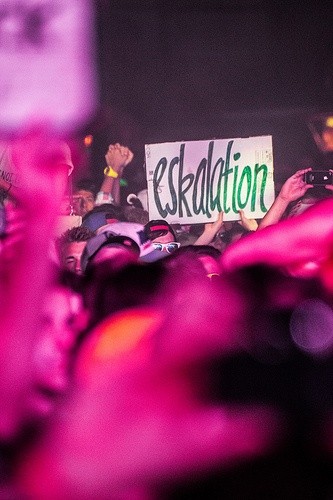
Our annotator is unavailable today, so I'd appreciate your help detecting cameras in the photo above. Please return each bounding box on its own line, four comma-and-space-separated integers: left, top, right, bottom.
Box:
193, 301, 332, 402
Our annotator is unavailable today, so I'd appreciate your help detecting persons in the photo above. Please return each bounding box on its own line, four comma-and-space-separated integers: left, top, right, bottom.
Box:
0, 0, 333, 499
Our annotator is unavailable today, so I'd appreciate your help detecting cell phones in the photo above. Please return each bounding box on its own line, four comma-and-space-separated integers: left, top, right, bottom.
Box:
303, 170, 333, 184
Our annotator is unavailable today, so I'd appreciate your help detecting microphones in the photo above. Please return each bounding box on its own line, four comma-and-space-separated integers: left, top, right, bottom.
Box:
127, 194, 143, 210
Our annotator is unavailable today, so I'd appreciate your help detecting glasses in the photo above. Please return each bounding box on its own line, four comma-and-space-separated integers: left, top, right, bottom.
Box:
210, 232, 230, 243
153, 241, 181, 254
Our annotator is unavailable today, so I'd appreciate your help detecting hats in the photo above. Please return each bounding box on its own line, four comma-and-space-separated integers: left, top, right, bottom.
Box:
80, 230, 141, 274
144, 220, 176, 241
